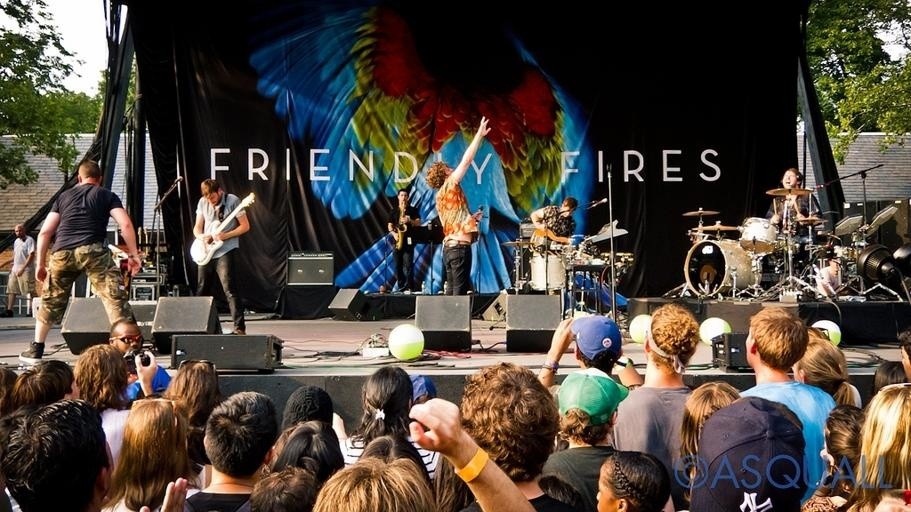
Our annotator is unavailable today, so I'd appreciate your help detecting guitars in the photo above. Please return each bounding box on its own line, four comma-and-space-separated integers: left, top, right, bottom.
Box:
190, 193, 255, 266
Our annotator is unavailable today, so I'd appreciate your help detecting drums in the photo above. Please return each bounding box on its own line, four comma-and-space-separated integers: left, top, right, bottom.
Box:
740, 217, 776, 253
530, 250, 567, 290
684, 240, 763, 295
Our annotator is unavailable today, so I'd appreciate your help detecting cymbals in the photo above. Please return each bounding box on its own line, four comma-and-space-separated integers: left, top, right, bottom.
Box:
767, 188, 812, 195
796, 218, 828, 223
502, 242, 533, 246
683, 211, 719, 215
693, 226, 739, 232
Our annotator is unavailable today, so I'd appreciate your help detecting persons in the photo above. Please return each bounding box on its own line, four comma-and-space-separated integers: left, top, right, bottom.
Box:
386, 189, 421, 293
763, 169, 823, 218
0, 298, 911, 512
20, 158, 144, 360
192, 178, 252, 336
814, 254, 845, 297
425, 114, 492, 296
529, 195, 578, 241
0, 220, 35, 317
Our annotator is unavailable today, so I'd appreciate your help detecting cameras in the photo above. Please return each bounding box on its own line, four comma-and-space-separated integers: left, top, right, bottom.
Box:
123, 349, 149, 374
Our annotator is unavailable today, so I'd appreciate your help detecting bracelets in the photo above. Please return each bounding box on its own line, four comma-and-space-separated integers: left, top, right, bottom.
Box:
127, 253, 138, 258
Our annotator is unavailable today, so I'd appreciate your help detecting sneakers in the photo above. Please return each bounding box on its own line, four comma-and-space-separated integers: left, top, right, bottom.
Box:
19, 342, 44, 364
0, 310, 14, 318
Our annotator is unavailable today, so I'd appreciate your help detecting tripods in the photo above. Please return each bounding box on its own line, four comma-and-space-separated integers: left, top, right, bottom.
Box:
364, 226, 401, 295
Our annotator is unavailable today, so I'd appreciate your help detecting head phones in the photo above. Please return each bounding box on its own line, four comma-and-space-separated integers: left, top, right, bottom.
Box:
796, 172, 803, 181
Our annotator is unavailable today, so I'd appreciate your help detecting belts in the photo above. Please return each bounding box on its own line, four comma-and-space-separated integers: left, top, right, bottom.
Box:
446, 240, 470, 245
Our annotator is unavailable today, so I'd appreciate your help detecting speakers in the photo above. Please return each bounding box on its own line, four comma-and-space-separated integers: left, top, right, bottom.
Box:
710, 333, 748, 367
506, 295, 561, 352
151, 297, 223, 355
285, 251, 335, 286
170, 334, 284, 370
60, 296, 157, 355
414, 295, 471, 352
327, 288, 366, 321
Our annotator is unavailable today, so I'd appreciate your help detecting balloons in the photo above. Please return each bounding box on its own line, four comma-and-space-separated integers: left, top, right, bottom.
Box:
698, 316, 733, 347
811, 320, 842, 347
386, 323, 426, 361
629, 313, 652, 346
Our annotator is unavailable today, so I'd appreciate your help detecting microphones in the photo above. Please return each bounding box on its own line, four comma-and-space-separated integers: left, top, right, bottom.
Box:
402, 201, 405, 210
174, 176, 183, 184
587, 198, 608, 210
809, 184, 824, 189
476, 205, 483, 225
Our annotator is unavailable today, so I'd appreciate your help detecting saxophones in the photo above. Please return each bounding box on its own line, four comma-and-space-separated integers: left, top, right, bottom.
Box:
392, 201, 407, 250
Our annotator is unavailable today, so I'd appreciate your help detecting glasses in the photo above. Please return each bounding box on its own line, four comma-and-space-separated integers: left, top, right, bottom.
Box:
109, 334, 142, 345
179, 359, 216, 377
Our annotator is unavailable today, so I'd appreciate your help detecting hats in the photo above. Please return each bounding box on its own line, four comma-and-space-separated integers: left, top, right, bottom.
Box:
409, 375, 436, 404
689, 397, 806, 512
558, 368, 629, 427
571, 316, 622, 361
